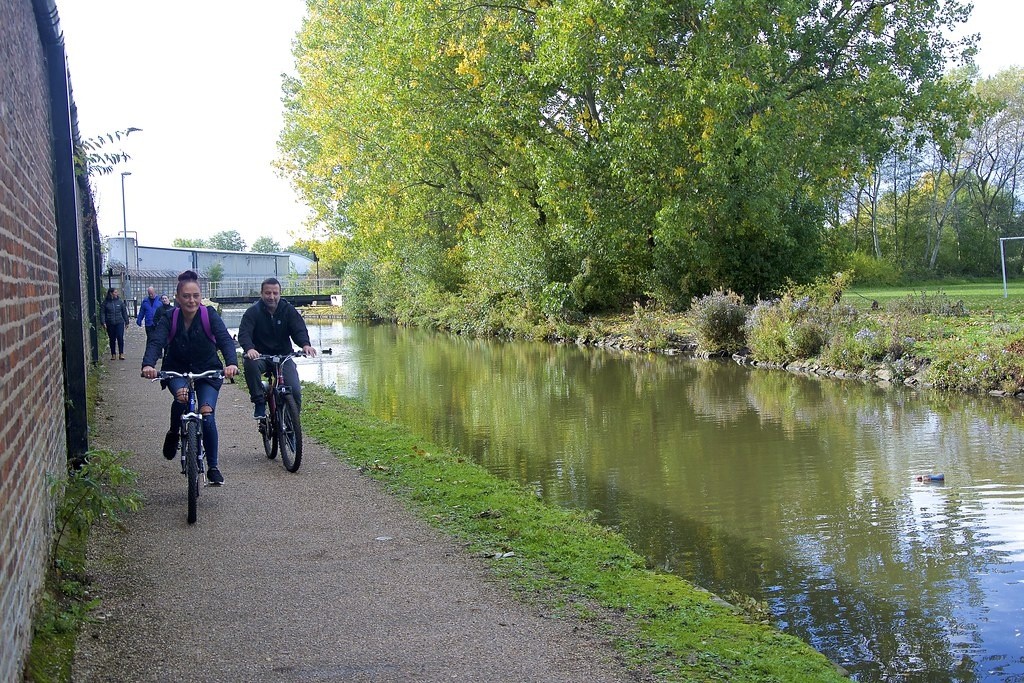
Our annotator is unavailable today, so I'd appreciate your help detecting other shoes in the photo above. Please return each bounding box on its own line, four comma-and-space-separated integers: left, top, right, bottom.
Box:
111, 354, 116, 360
118, 353, 125, 360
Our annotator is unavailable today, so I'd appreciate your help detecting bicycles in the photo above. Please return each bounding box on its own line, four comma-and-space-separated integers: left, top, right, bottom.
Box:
242, 348, 333, 473
139, 369, 239, 524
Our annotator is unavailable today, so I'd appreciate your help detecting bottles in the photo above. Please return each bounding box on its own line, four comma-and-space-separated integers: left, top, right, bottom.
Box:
914, 474, 944, 482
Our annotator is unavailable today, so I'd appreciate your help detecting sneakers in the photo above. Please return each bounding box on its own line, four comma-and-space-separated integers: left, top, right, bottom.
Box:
163, 429, 180, 460
207, 466, 224, 485
253, 402, 266, 419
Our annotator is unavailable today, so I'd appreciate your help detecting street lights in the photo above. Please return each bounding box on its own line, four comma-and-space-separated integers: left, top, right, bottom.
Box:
121, 171, 132, 298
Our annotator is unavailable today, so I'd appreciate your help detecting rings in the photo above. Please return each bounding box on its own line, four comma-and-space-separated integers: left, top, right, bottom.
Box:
147, 374, 150, 378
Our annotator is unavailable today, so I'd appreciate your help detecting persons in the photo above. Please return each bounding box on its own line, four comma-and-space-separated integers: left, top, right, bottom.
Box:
136, 286, 175, 359
100, 287, 129, 360
141, 269, 240, 486
238, 277, 317, 452
